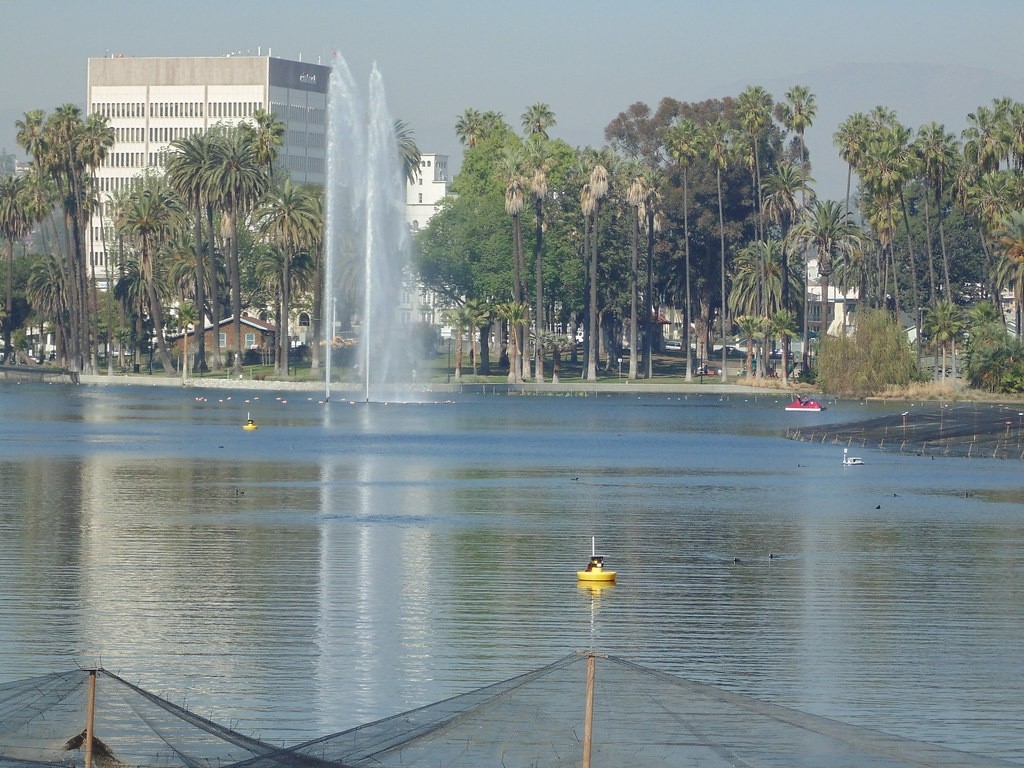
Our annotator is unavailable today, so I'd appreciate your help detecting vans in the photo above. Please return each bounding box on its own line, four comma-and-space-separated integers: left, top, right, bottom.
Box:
567, 330, 591, 345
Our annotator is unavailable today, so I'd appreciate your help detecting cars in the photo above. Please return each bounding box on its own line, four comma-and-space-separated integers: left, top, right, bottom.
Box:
665, 340, 682, 350
715, 347, 746, 359
768, 348, 794, 360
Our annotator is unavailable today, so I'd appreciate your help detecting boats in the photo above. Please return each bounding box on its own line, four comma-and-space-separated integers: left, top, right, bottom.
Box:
577, 535, 617, 581
242, 411, 257, 433
842, 447, 865, 465
785, 397, 822, 411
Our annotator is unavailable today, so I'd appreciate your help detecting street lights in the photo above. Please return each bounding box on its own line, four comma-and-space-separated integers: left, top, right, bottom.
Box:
699, 342, 705, 384
445, 337, 453, 382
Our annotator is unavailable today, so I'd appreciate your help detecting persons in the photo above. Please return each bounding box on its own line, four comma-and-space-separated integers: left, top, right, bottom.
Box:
801, 395, 810, 406
